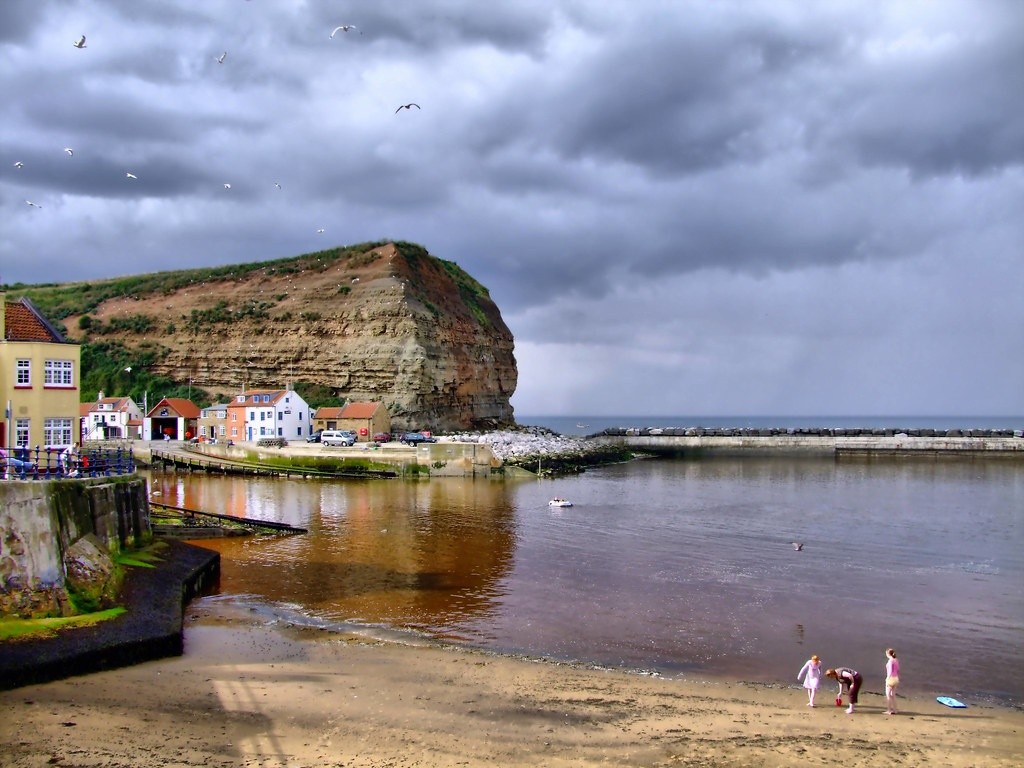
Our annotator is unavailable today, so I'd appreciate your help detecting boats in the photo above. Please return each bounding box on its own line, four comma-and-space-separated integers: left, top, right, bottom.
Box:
548, 498, 573, 507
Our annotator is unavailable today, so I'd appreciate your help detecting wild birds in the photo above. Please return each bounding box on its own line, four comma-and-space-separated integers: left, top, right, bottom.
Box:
273, 182, 281, 190
124, 366, 132, 373
23, 199, 43, 209
316, 228, 325, 234
126, 172, 138, 180
209, 51, 228, 64
223, 183, 232, 191
13, 161, 25, 169
327, 24, 363, 41
786, 541, 805, 552
394, 102, 422, 116
73, 34, 88, 48
65, 147, 74, 157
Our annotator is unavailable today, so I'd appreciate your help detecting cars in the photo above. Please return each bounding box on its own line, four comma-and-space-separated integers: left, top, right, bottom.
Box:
400, 432, 437, 447
190, 438, 200, 446
373, 432, 391, 443
343, 430, 358, 442
205, 438, 218, 445
305, 433, 321, 443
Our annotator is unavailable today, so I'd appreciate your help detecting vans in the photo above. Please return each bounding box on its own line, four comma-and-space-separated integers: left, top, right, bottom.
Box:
321, 431, 354, 447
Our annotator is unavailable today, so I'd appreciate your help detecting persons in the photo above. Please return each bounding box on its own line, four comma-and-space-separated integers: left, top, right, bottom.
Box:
797, 655, 823, 707
825, 667, 864, 714
881, 649, 901, 715
0, 449, 39, 473
165, 434, 170, 446
79, 454, 90, 477
60, 441, 80, 475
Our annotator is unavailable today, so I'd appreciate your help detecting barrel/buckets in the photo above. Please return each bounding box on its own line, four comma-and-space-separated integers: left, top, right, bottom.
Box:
836, 699, 842, 706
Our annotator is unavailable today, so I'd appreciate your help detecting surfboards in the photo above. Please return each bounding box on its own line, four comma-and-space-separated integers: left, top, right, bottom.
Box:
937, 696, 967, 708
